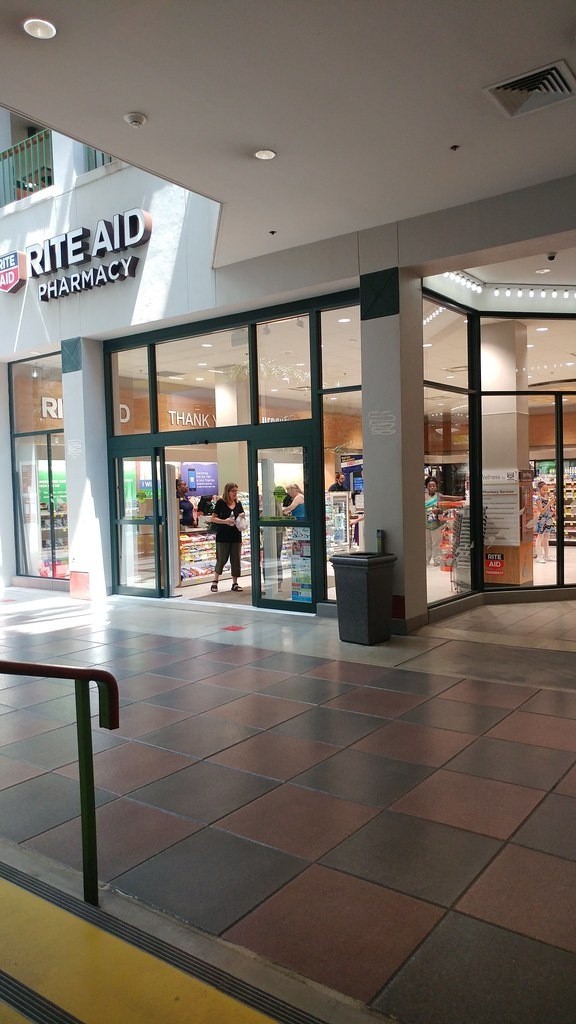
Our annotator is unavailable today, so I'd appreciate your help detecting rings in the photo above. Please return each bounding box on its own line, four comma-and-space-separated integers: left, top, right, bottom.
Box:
193, 520, 195, 521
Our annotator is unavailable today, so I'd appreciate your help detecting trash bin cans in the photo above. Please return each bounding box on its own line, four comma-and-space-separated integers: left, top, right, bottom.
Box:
328, 551, 398, 645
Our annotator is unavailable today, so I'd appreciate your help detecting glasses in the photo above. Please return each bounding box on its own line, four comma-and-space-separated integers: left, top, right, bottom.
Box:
230, 489, 239, 494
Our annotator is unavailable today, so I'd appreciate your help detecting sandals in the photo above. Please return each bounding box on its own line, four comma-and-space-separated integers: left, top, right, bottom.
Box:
231, 583, 243, 592
210, 580, 219, 592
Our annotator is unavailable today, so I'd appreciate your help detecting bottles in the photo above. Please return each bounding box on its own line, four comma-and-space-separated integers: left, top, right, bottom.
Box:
229, 511, 235, 526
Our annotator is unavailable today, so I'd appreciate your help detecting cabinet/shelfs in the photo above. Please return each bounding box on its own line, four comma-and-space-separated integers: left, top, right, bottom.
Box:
288, 492, 350, 588
536, 474, 576, 545
177, 493, 252, 589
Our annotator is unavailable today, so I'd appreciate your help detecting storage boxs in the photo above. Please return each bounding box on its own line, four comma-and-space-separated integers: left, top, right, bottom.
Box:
138, 498, 160, 535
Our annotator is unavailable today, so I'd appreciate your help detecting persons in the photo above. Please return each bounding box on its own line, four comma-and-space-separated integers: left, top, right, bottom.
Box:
351, 515, 364, 525
536, 482, 556, 562
425, 477, 463, 566
211, 482, 245, 592
176, 479, 196, 528
327, 473, 348, 490
198, 495, 214, 516
282, 484, 304, 517
259, 491, 283, 592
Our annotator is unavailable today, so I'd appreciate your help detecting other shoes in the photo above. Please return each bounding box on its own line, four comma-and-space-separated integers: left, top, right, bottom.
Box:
433, 562, 438, 566
535, 555, 546, 563
543, 555, 557, 561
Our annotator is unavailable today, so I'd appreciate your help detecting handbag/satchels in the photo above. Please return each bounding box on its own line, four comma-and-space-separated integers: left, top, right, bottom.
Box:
208, 522, 218, 534
